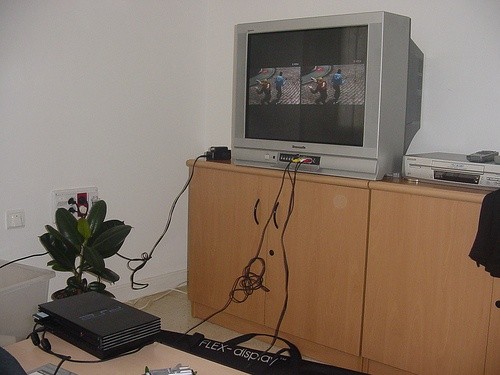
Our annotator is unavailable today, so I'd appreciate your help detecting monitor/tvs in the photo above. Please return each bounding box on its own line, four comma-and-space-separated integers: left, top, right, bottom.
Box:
230, 11, 424, 181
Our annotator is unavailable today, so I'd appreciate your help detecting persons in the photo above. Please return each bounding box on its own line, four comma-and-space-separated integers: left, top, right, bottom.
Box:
330, 68, 344, 102
275, 71, 286, 99
254, 79, 272, 105
308, 77, 328, 104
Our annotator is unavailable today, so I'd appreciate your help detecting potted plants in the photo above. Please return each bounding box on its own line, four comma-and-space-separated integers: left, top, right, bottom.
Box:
38, 199, 133, 303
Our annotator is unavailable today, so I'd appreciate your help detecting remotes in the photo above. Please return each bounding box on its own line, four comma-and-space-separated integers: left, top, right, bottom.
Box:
466, 150, 499, 163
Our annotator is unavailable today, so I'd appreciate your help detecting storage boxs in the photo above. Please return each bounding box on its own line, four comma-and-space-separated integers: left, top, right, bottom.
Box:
0, 258, 57, 335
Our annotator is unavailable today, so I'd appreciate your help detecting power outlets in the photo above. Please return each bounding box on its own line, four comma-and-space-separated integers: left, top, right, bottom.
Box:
51, 187, 98, 222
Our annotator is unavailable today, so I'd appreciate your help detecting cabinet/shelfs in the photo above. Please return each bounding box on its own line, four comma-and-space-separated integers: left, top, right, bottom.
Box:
184, 157, 500, 375
